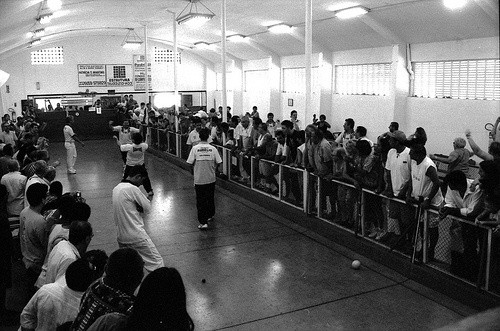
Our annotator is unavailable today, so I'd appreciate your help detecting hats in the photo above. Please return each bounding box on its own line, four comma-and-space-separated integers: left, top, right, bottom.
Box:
388, 130, 407, 142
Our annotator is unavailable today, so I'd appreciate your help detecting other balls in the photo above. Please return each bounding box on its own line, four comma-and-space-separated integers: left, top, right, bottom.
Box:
351, 259, 361, 269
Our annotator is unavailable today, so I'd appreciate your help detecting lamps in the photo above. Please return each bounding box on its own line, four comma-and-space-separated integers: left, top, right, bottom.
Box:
120, 28, 143, 50
226, 34, 245, 41
30, 0, 53, 45
176, 0, 216, 27
194, 41, 208, 49
334, 5, 370, 20
268, 23, 290, 33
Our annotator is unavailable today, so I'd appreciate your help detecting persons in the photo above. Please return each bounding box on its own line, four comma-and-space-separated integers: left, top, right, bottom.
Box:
113, 132, 154, 195
0, 99, 195, 331
64, 117, 85, 174
109, 119, 144, 172
186, 127, 222, 228
110, 95, 500, 293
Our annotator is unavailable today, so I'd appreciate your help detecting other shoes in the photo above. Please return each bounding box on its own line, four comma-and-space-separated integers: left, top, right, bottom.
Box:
197, 223, 208, 228
375, 231, 389, 240
368, 231, 377, 238
67, 167, 77, 173
